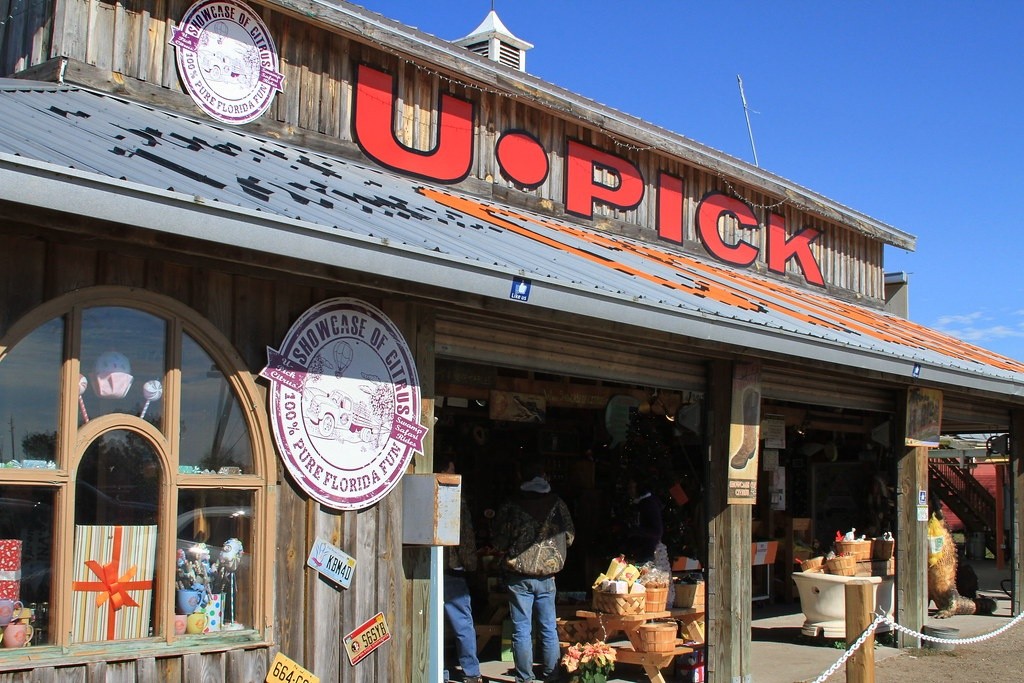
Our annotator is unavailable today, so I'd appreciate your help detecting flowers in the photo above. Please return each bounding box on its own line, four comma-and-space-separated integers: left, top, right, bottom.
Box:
559, 639, 618, 683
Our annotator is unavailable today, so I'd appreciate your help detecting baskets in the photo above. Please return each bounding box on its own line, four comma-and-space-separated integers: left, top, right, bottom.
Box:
591, 590, 647, 616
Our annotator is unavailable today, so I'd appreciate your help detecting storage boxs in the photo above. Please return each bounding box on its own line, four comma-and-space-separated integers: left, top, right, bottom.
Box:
194, 593, 226, 632
674, 650, 704, 683
0, 539, 23, 609
73, 524, 157, 643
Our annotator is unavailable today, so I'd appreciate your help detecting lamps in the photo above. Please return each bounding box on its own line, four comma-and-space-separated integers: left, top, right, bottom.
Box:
475, 397, 489, 407
991, 434, 1010, 455
797, 411, 812, 437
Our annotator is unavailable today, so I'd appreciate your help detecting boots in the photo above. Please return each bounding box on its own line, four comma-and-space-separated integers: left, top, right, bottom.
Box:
730, 387, 759, 469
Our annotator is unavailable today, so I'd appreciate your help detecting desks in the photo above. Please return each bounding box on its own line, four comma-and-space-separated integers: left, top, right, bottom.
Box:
576, 607, 705, 683
476, 593, 512, 657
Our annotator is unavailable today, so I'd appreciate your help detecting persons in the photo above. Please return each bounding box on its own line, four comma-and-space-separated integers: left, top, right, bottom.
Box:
492, 463, 575, 683
434, 457, 489, 683
621, 476, 664, 559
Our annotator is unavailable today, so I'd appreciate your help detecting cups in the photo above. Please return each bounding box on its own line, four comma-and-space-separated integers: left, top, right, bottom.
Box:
186, 613, 210, 634
3, 623, 34, 648
175, 614, 187, 635
177, 589, 203, 615
0, 599, 24, 626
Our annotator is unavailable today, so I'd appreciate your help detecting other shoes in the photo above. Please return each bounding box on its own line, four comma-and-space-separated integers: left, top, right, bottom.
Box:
461, 675, 489, 683
